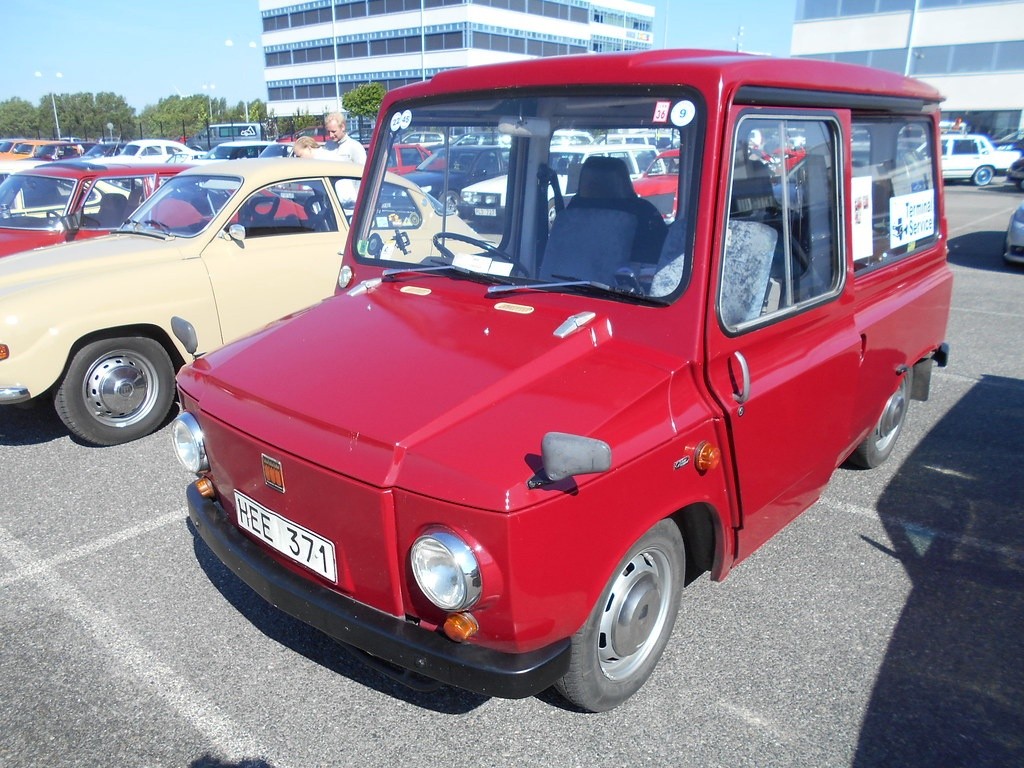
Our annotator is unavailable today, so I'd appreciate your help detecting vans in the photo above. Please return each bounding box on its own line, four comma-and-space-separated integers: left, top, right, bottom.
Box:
185, 123, 267, 151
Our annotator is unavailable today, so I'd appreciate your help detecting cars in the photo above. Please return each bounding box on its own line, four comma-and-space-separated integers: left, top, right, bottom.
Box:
1003, 201, 1024, 266
170, 49, 955, 708
345, 128, 377, 145
1007, 155, 1024, 190
275, 125, 334, 144
0, 159, 498, 447
382, 148, 512, 213
392, 128, 683, 150
1, 160, 310, 260
766, 119, 1024, 188
1, 138, 209, 187
169, 141, 448, 195
634, 146, 774, 226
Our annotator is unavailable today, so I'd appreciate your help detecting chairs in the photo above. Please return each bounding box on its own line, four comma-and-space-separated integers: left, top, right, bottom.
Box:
303, 194, 336, 232
538, 156, 663, 284
121, 188, 144, 225
731, 164, 804, 300
95, 193, 127, 229
247, 196, 280, 228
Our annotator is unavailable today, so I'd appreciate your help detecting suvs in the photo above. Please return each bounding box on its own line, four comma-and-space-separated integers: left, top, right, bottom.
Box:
457, 143, 665, 234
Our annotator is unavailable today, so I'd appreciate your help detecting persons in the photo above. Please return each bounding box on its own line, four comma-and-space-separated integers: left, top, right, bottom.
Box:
293, 136, 358, 231
321, 113, 367, 191
